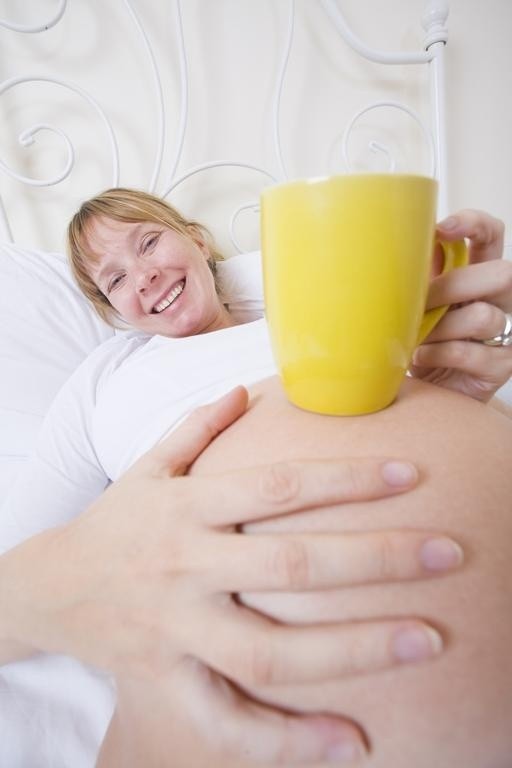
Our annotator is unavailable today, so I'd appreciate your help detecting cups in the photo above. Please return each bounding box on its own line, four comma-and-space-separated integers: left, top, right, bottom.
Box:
258, 171, 469, 420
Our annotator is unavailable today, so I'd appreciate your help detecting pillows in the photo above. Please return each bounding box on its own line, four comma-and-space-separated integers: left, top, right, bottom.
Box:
0, 247, 255, 553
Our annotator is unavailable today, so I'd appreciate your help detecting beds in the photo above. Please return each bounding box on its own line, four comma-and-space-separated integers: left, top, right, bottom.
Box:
0, 1, 460, 767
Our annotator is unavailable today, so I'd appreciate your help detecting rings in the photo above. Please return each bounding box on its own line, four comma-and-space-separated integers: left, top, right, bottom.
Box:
482, 312, 511, 346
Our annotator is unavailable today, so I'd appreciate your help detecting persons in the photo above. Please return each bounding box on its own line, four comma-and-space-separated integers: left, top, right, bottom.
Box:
0, 183, 511, 768
